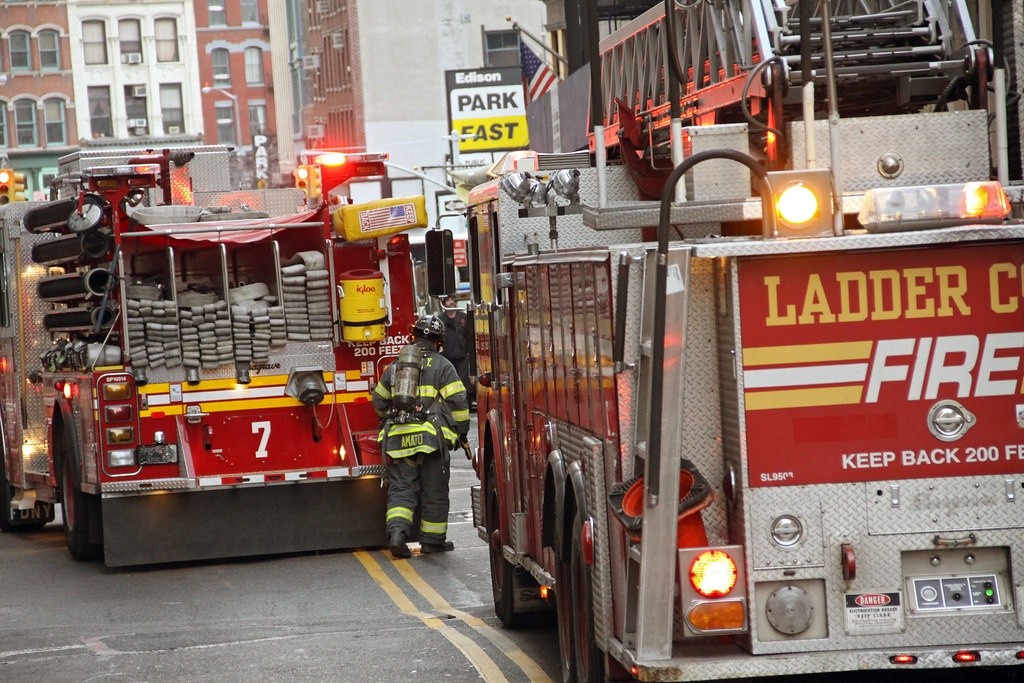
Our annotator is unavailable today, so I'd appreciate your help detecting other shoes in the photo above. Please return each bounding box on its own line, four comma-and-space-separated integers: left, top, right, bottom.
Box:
389, 532, 411, 557
420, 541, 454, 553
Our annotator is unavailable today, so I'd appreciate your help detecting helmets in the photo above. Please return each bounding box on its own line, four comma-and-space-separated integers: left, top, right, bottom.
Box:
415, 315, 445, 335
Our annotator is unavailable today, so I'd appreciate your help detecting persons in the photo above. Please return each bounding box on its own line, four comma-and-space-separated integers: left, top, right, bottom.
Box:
436, 295, 479, 408
372, 314, 470, 558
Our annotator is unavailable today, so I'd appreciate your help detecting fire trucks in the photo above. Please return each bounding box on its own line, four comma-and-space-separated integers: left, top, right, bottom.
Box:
423, 0, 1024, 682
0, 132, 429, 567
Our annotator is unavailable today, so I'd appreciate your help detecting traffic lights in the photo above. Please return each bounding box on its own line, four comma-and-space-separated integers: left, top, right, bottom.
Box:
13, 173, 26, 203
308, 164, 322, 199
294, 167, 309, 198
0, 168, 15, 206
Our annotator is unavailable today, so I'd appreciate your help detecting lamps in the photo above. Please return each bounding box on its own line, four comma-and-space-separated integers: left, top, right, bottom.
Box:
501, 169, 582, 247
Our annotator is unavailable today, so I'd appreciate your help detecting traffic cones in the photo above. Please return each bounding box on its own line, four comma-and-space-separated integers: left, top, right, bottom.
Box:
607, 456, 715, 592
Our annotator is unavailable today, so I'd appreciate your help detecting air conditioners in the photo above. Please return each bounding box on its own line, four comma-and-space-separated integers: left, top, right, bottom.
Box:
306, 124, 324, 139
136, 118, 147, 127
128, 53, 142, 63
302, 55, 320, 70
132, 86, 146, 97
168, 126, 179, 133
332, 34, 343, 49
